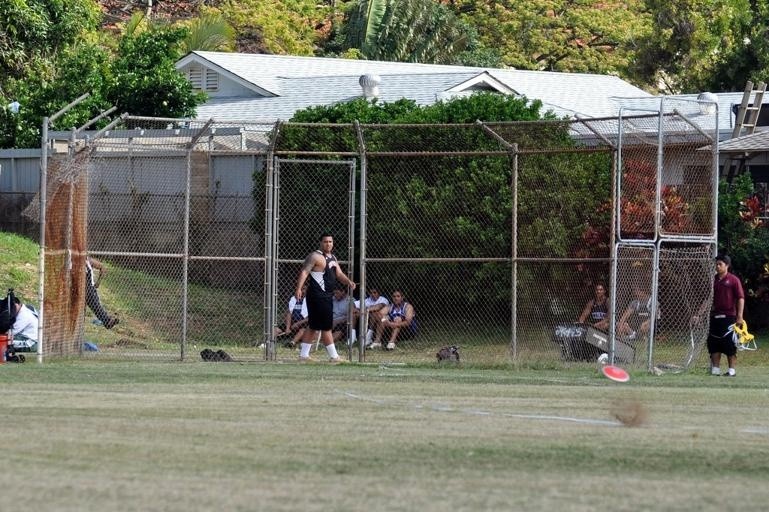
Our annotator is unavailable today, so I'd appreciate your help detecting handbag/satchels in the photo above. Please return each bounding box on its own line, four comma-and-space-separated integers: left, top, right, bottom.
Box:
437, 345, 459, 361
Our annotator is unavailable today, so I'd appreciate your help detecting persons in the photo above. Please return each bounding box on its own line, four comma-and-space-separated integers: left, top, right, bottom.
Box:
691, 254, 745, 377
579, 281, 662, 342
259, 282, 418, 353
7, 297, 38, 352
85, 256, 119, 329
295, 233, 356, 363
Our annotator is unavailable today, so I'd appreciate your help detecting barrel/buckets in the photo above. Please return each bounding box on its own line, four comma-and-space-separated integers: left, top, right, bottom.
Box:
0, 334, 7, 363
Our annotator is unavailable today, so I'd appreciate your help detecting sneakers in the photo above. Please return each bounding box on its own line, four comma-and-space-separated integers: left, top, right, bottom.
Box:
284, 341, 294, 349
625, 331, 639, 339
387, 342, 395, 351
299, 357, 320, 365
711, 367, 736, 377
345, 338, 372, 346
330, 357, 345, 364
103, 317, 120, 330
367, 343, 381, 351
